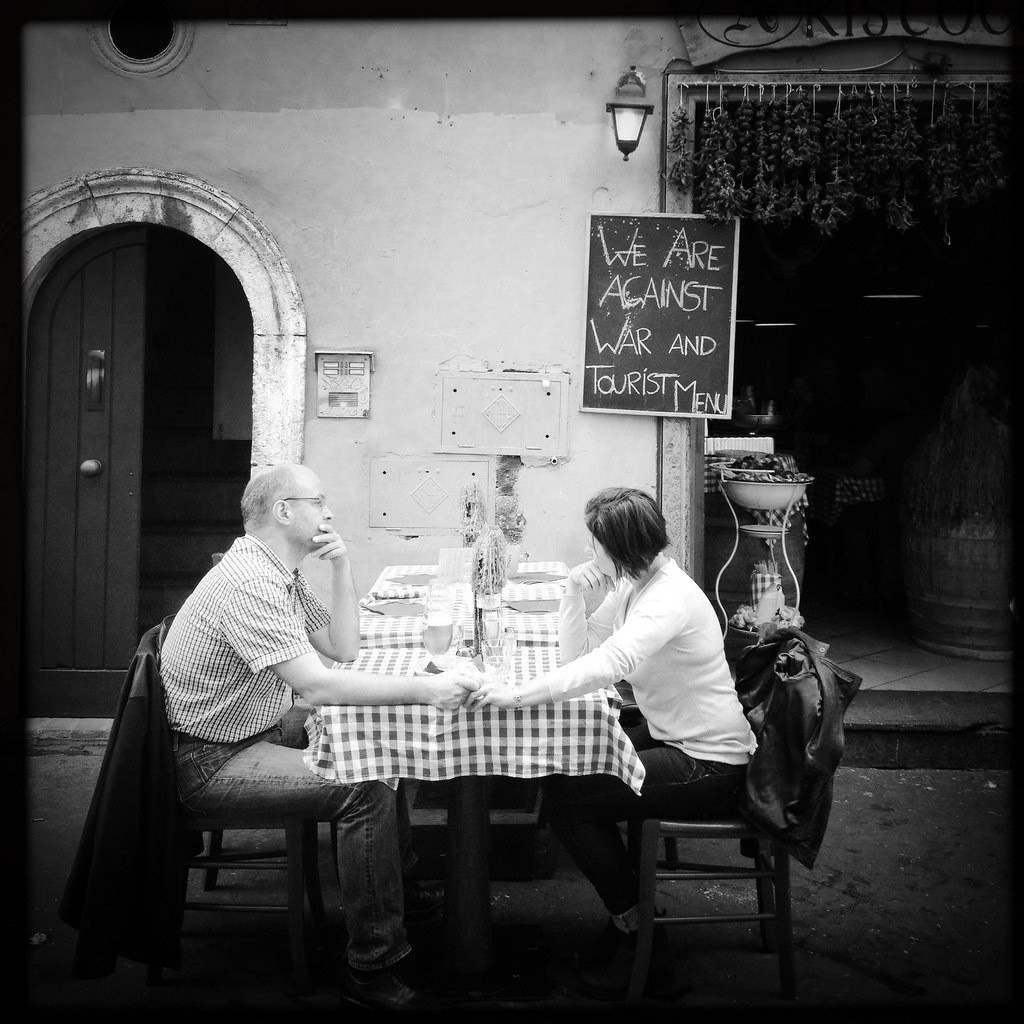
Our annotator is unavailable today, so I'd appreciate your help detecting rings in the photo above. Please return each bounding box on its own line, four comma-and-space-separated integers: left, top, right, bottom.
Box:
476, 690, 480, 697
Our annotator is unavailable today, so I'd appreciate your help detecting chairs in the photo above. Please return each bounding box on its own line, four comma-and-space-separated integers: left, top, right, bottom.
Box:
612, 625, 805, 1003
131, 623, 332, 999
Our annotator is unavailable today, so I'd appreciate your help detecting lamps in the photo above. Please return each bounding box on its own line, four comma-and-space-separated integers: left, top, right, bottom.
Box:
604, 62, 656, 162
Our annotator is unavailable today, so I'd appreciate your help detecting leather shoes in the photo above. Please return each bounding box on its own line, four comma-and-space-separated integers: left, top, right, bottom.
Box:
567, 908, 668, 976
340, 962, 457, 1021
575, 932, 689, 1002
403, 875, 445, 920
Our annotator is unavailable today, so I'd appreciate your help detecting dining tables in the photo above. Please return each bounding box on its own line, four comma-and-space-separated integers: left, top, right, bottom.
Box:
323, 560, 615, 1001
812, 466, 883, 597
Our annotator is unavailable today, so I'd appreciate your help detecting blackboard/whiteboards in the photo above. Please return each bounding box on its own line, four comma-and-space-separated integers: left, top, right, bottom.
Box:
579, 210, 740, 420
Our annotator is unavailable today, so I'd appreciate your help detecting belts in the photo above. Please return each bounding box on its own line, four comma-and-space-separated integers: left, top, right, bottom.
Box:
168, 729, 204, 745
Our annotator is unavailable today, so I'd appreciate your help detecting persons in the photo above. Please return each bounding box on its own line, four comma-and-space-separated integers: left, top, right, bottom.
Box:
160, 464, 484, 1013
462, 487, 758, 1003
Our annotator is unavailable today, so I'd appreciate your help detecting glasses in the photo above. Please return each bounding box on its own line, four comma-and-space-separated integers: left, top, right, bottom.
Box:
283, 497, 326, 513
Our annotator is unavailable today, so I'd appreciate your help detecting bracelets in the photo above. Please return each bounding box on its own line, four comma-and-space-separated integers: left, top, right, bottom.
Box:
513, 686, 523, 711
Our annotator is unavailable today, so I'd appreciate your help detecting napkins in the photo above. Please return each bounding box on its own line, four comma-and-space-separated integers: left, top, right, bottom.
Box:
385, 574, 438, 588
365, 602, 426, 617
508, 572, 567, 586
506, 598, 561, 616
